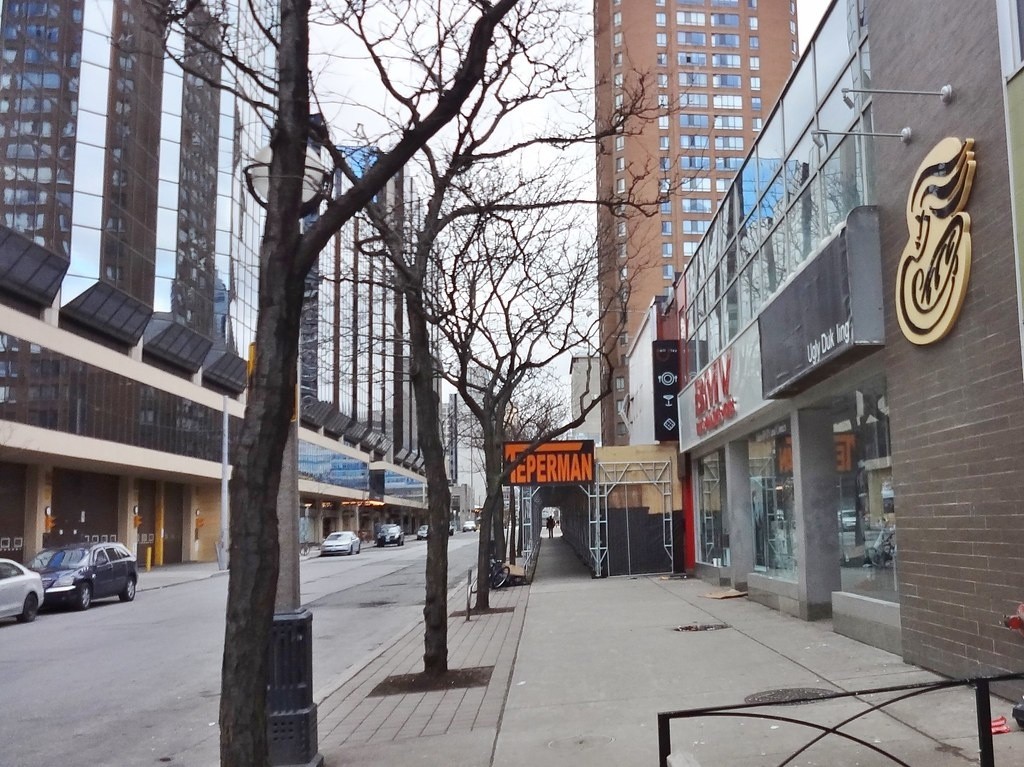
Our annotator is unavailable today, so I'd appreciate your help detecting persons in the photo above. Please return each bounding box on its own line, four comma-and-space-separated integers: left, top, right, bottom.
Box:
546, 516, 555, 539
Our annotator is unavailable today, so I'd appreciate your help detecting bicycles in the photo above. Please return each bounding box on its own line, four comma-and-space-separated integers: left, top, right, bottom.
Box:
470, 560, 510, 593
865, 532, 895, 568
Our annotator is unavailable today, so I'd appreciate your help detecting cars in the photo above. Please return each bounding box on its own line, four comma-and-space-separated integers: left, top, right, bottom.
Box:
24, 542, 136, 610
449, 522, 455, 535
417, 525, 428, 539
321, 531, 360, 556
838, 510, 870, 532
463, 521, 476, 532
0, 559, 45, 623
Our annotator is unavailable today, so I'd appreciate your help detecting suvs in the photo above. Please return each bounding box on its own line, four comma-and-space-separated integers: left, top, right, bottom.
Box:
377, 525, 404, 547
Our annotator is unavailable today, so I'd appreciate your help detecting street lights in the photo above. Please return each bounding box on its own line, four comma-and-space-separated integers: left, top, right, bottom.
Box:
241, 139, 331, 767
